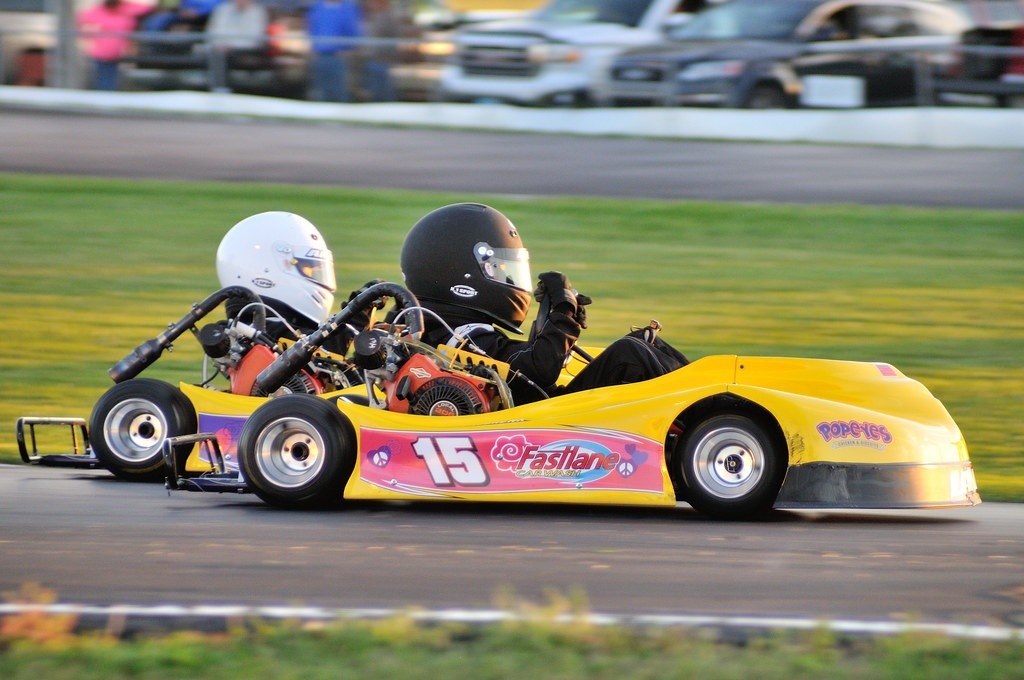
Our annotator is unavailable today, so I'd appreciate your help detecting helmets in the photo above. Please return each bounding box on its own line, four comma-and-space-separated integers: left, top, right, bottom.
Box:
399, 202, 532, 334
216, 211, 337, 325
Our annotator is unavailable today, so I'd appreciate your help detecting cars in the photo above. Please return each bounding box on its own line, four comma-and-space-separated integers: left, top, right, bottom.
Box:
594, 0, 975, 106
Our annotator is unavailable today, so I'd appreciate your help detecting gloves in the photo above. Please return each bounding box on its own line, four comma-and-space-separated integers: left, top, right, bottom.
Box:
341, 278, 390, 334
534, 271, 592, 328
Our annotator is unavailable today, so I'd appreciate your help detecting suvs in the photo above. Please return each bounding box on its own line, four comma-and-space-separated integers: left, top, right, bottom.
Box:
444, 0, 708, 109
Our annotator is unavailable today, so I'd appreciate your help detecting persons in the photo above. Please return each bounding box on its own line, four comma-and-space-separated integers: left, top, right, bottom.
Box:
74, 0, 161, 91
305, 0, 400, 102
216, 211, 400, 394
400, 203, 690, 407
206, 0, 269, 93
